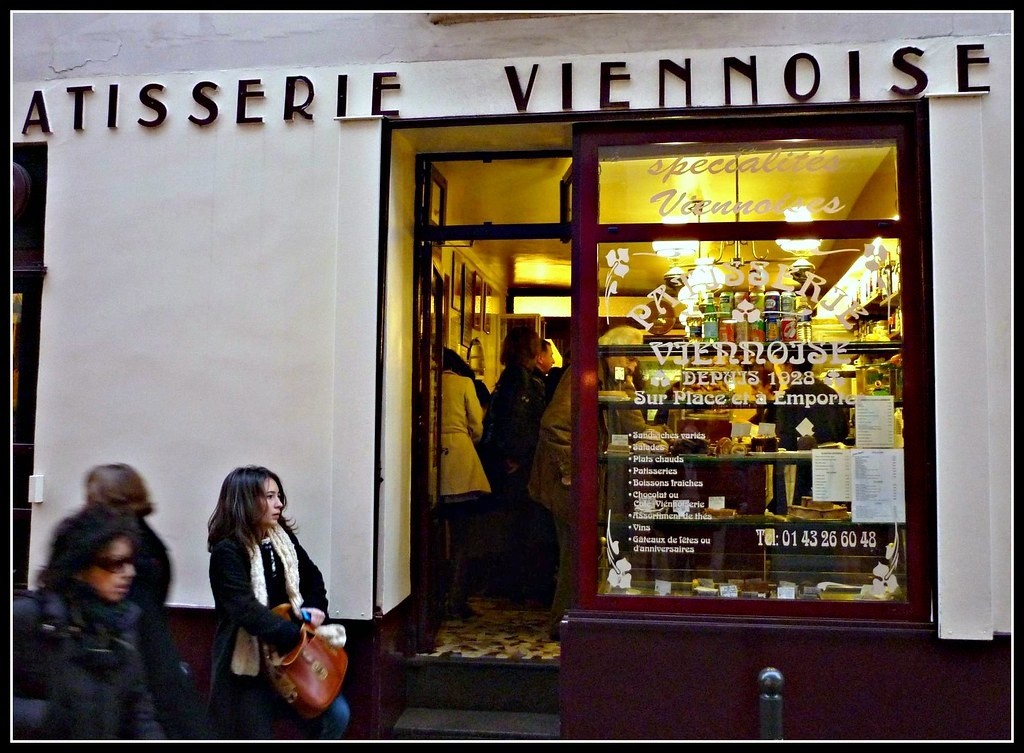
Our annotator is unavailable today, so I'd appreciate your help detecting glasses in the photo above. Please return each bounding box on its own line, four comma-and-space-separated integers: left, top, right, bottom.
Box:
91, 555, 132, 573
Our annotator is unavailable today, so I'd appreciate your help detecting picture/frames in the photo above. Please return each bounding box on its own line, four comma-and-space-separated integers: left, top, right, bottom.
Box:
430, 164, 448, 246
483, 281, 492, 335
462, 263, 473, 350
472, 270, 483, 331
432, 219, 442, 266
452, 251, 462, 312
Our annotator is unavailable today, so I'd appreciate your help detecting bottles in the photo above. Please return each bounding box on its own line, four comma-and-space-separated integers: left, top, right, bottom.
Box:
702, 292, 718, 342
797, 298, 812, 341
686, 305, 703, 342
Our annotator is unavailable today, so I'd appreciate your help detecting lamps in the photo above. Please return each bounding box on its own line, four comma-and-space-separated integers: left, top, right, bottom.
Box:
661, 154, 814, 288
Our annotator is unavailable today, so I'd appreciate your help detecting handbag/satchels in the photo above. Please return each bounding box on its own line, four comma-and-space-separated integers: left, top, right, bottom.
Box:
262, 603, 350, 718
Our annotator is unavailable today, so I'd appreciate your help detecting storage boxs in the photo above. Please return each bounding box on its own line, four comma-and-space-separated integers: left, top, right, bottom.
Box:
635, 464, 772, 511
769, 528, 896, 584
628, 527, 765, 582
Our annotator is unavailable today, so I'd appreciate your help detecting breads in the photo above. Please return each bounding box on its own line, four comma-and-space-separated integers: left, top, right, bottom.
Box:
787, 496, 847, 520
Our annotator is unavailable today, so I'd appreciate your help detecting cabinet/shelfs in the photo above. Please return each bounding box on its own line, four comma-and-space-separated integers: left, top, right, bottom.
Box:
601, 340, 902, 585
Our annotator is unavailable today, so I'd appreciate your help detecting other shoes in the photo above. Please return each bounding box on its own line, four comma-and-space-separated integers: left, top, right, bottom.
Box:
440, 610, 460, 628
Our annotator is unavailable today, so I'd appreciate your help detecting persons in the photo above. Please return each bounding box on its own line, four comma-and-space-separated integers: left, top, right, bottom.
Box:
13, 506, 169, 740
437, 324, 678, 642
205, 467, 350, 740
37, 464, 204, 738
740, 352, 851, 521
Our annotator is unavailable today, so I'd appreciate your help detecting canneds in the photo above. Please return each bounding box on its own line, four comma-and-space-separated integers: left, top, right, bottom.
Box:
718, 267, 797, 343
